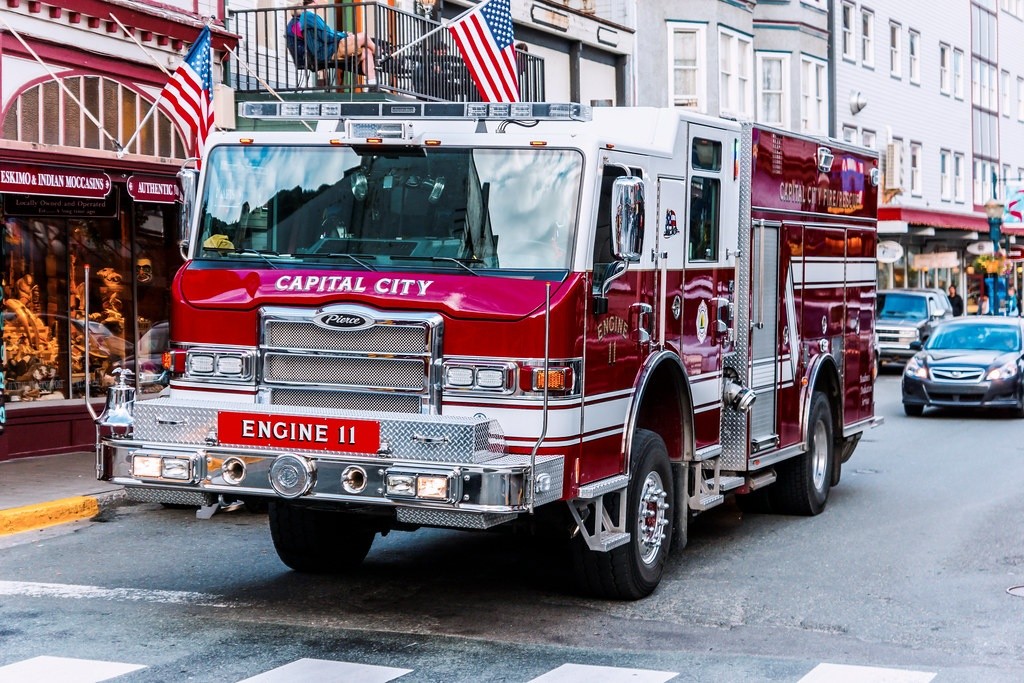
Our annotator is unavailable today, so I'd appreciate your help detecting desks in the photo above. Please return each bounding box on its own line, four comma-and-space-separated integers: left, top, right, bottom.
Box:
407, 54, 462, 97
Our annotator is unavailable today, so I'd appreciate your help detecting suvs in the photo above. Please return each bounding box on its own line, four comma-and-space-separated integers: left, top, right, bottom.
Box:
877, 288, 955, 372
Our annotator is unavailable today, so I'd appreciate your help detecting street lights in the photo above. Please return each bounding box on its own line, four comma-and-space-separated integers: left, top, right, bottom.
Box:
984, 199, 1007, 316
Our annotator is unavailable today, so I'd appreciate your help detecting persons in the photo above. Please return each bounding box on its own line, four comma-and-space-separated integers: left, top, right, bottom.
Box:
1006, 286, 1021, 318
981, 293, 991, 315
286, 0, 395, 94
947, 284, 964, 317
413, 41, 457, 102
514, 41, 529, 74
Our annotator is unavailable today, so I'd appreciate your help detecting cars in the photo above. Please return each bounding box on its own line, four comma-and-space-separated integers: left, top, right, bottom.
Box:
2, 311, 135, 388
900, 314, 1024, 416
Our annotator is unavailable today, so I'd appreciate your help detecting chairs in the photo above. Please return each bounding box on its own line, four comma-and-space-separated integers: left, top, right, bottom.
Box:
371, 37, 412, 93
280, 32, 367, 93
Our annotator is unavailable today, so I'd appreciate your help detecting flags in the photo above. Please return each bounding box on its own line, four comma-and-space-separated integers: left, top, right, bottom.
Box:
447, 0, 520, 102
159, 26, 213, 170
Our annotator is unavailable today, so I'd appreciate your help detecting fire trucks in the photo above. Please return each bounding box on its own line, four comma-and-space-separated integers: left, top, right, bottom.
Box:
85, 3, 880, 600
